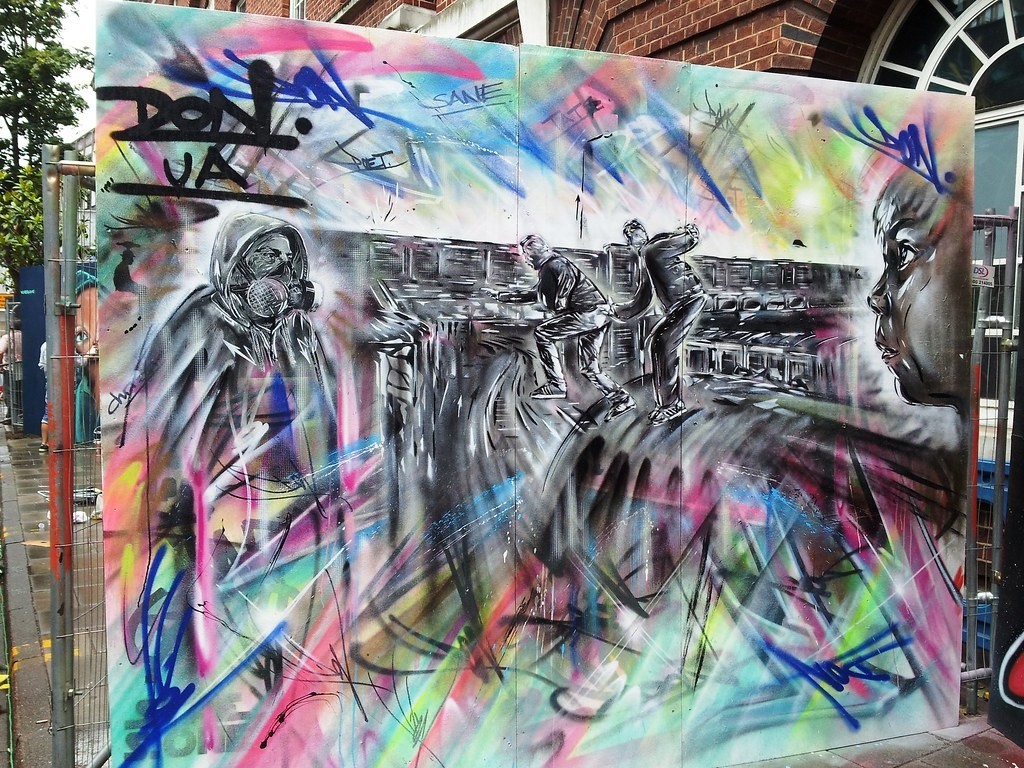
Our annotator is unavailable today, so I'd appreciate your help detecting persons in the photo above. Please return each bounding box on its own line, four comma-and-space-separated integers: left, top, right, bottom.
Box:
0, 318, 22, 425
38, 339, 99, 452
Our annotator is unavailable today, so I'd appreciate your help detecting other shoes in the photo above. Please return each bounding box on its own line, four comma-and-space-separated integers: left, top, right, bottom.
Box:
39, 444, 48, 451
3, 420, 11, 425
